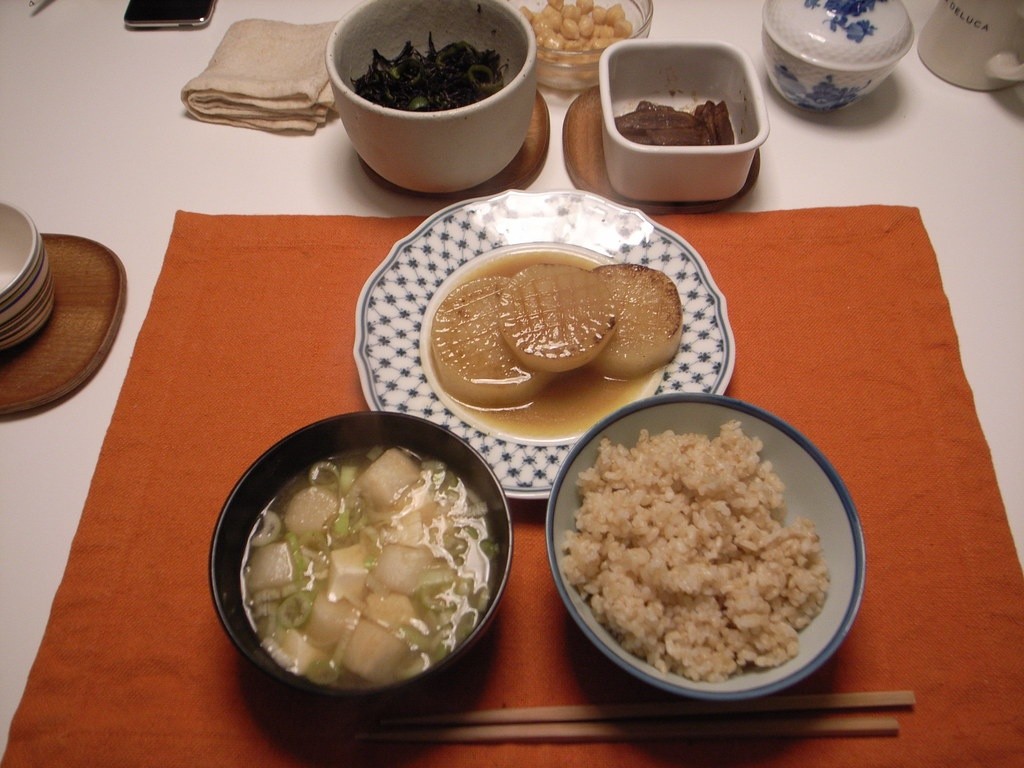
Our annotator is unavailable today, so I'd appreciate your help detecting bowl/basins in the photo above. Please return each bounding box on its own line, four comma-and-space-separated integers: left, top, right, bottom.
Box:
545, 393, 866, 702
324, 0, 539, 193
504, 0, 654, 93
207, 410, 515, 702
599, 37, 768, 203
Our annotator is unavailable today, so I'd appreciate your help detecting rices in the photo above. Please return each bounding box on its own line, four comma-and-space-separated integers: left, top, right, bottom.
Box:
560, 420, 830, 684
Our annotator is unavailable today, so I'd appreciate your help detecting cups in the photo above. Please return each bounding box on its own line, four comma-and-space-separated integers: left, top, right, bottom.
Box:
761, 0, 916, 115
917, 0, 1023, 93
0, 201, 55, 355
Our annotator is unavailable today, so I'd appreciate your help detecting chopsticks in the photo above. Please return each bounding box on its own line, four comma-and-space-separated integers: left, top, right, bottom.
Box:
351, 688, 917, 745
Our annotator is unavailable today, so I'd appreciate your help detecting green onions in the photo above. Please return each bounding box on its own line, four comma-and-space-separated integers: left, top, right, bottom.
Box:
248, 447, 501, 685
384, 41, 506, 111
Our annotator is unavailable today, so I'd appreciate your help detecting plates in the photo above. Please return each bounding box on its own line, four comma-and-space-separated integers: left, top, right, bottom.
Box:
352, 188, 735, 499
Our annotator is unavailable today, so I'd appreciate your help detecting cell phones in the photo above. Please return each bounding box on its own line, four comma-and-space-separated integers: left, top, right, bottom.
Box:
123, 0, 213, 30
